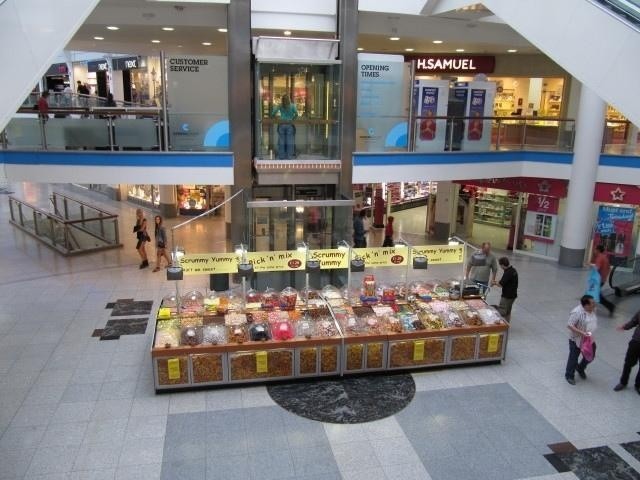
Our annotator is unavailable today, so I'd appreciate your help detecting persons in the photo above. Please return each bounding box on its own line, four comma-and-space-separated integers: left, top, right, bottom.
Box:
382, 215, 394, 247
612, 308, 640, 396
306, 205, 325, 250
353, 208, 368, 248
586, 244, 616, 319
468, 111, 483, 141
489, 255, 519, 324
421, 109, 437, 141
132, 208, 151, 271
270, 94, 299, 161
465, 241, 498, 297
563, 294, 598, 386
152, 215, 173, 273
35, 80, 118, 125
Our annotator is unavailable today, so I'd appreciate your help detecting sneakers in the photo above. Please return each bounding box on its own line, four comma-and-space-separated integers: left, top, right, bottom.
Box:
576, 365, 586, 379
152, 266, 160, 273
565, 373, 575, 385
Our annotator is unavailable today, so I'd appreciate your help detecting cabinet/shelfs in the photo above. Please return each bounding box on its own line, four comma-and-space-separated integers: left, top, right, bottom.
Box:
472, 192, 513, 228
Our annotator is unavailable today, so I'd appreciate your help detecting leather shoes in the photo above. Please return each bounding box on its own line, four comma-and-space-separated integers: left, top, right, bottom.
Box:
614, 382, 627, 391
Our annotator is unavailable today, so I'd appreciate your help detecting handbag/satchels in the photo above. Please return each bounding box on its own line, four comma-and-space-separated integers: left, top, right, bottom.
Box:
579, 334, 594, 362
585, 266, 601, 304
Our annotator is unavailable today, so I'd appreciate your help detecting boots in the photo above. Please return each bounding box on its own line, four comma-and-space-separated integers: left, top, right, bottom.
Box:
140, 260, 149, 269
